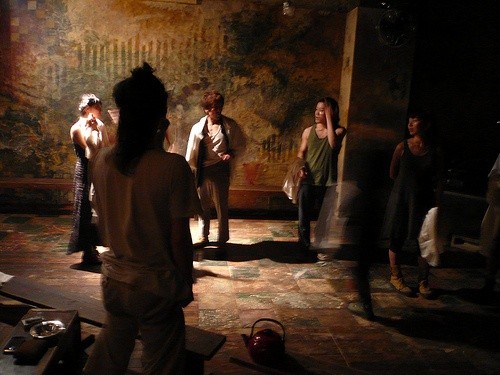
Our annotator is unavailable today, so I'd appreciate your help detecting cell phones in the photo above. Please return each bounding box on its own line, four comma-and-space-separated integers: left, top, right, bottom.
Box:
3, 336, 26, 353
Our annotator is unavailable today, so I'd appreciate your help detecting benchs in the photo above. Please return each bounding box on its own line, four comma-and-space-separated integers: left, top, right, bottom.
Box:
0, 277, 226, 375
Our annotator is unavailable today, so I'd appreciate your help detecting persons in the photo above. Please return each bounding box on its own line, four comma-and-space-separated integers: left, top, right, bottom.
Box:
478, 148, 500, 299
185, 91, 244, 258
294, 97, 347, 261
384, 108, 442, 300
66, 93, 112, 265
81, 60, 203, 375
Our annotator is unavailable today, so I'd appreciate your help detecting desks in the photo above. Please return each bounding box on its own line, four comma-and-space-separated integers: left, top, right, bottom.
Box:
0, 309, 80, 375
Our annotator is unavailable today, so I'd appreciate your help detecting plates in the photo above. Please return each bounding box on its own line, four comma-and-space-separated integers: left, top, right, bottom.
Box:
30, 321, 64, 339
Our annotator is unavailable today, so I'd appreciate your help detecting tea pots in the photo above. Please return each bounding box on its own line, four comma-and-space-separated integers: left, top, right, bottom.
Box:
240, 318, 286, 367
